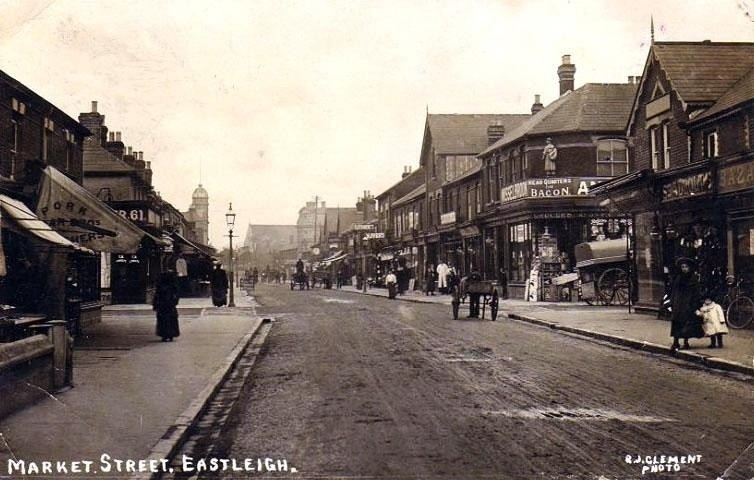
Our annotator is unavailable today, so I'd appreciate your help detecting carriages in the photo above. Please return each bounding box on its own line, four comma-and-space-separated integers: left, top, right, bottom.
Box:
449, 266, 500, 322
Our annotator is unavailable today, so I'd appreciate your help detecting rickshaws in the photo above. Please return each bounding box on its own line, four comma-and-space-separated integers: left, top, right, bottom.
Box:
572, 236, 635, 306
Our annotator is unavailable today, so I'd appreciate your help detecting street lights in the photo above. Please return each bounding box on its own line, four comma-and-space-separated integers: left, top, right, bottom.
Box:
224, 202, 242, 309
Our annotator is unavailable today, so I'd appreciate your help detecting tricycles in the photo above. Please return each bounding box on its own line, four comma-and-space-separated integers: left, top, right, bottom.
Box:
289, 271, 311, 291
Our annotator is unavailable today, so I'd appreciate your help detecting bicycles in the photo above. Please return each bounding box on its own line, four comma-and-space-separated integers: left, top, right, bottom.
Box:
717, 276, 754, 332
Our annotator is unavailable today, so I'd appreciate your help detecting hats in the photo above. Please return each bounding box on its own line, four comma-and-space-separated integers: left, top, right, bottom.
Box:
676, 257, 694, 267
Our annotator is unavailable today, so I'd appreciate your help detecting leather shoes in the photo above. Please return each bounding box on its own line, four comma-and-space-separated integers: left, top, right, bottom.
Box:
672, 342, 723, 349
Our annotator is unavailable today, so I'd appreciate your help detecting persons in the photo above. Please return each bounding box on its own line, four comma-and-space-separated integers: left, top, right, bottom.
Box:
152, 270, 181, 342
542, 136, 557, 178
210, 259, 483, 319
670, 256, 705, 349
696, 296, 729, 349
